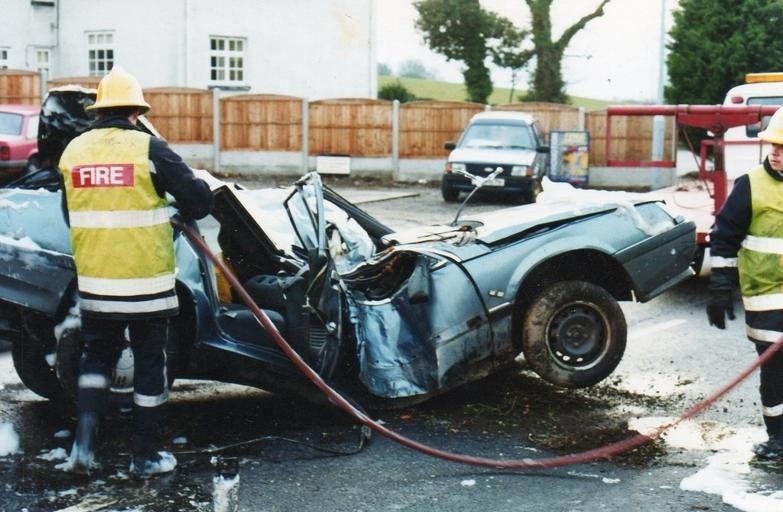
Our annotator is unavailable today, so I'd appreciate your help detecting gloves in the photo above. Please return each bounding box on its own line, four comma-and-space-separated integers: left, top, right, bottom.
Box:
704, 291, 736, 328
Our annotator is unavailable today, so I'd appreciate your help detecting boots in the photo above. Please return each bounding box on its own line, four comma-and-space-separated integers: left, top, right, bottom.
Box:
749, 429, 783, 459
69, 410, 100, 475
129, 424, 178, 482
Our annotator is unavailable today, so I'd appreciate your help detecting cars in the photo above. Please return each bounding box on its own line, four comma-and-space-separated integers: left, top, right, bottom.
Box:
0, 103, 42, 177
0, 82, 696, 412
439, 109, 550, 206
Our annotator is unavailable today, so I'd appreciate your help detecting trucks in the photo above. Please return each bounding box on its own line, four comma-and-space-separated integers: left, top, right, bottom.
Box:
605, 73, 783, 278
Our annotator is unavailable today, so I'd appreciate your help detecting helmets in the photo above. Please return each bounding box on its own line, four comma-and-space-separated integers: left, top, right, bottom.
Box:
84, 68, 151, 115
756, 107, 783, 147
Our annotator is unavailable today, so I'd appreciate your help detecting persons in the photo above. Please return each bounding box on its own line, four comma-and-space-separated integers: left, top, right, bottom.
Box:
57, 69, 212, 478
706, 106, 783, 459
